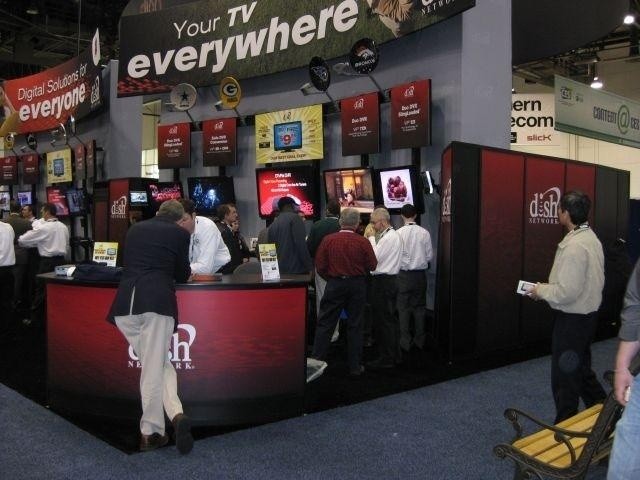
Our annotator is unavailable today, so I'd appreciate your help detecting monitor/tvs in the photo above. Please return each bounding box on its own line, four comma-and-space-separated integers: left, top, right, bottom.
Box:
17, 191, 33, 206
0, 190, 11, 212
187, 175, 234, 214
129, 190, 150, 207
273, 121, 302, 153
64, 187, 91, 217
52, 158, 65, 178
254, 165, 321, 219
147, 181, 184, 206
45, 186, 71, 217
323, 166, 380, 217
377, 164, 425, 215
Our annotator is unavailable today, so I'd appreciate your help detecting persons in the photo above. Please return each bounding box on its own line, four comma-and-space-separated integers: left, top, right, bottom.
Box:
310, 209, 378, 380
0, 208, 16, 342
294, 210, 306, 224
257, 210, 279, 257
522, 190, 607, 426
230, 215, 250, 257
396, 204, 432, 355
106, 199, 194, 455
368, 208, 403, 362
177, 198, 237, 274
18, 202, 71, 325
214, 203, 237, 274
22, 204, 37, 224
308, 200, 345, 345
5, 200, 33, 312
606, 257, 640, 480
268, 197, 313, 274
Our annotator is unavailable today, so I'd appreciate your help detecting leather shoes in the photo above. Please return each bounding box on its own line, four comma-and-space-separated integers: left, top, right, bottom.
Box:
171, 413, 193, 454
140, 435, 168, 452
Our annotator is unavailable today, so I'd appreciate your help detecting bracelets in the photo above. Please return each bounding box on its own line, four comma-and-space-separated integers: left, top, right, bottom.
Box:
613, 368, 629, 373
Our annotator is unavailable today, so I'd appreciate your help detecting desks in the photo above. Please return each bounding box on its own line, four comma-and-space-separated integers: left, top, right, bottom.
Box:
36, 263, 313, 429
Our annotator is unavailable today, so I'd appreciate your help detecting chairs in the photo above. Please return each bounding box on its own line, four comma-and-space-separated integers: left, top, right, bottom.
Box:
494, 356, 639, 480
